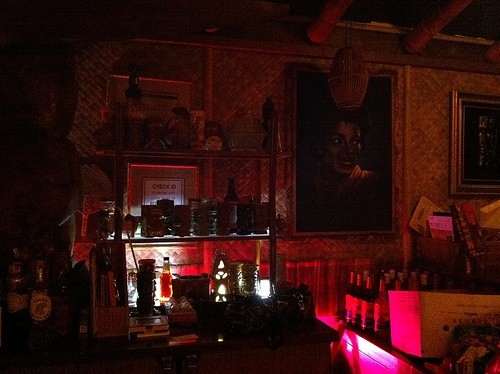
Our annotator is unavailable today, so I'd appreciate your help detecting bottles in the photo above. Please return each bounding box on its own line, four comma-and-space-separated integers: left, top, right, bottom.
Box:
161, 257, 173, 306
188, 196, 202, 233
211, 251, 228, 302
0, 244, 91, 358
224, 177, 240, 232
345, 268, 458, 343
247, 196, 256, 233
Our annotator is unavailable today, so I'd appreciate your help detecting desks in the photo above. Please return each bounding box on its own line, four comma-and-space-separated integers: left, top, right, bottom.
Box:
337, 311, 432, 374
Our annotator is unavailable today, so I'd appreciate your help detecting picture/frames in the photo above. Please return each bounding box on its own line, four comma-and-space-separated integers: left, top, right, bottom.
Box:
449, 89, 500, 198
286, 62, 401, 243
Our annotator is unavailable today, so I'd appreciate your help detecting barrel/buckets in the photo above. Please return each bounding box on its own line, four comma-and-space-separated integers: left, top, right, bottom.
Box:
228, 263, 261, 296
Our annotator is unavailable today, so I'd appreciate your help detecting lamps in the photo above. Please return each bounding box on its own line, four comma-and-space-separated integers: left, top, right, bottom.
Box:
329, 20, 369, 109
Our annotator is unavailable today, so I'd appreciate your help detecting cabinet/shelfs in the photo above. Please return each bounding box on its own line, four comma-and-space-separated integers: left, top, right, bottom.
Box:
79, 95, 277, 305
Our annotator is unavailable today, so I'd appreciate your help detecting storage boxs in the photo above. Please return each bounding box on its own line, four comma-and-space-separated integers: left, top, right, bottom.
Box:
417, 225, 500, 284
389, 289, 500, 358
